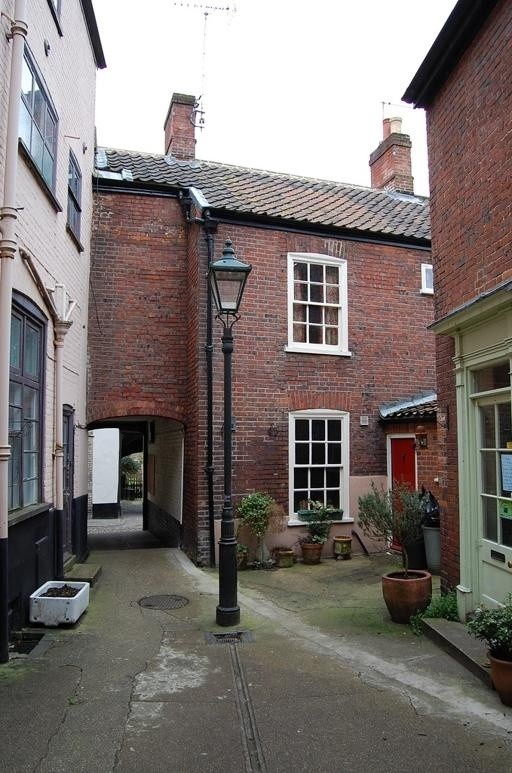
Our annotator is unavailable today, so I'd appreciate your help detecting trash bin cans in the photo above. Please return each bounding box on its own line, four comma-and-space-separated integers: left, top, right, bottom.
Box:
421, 523, 441, 574
121, 488, 136, 501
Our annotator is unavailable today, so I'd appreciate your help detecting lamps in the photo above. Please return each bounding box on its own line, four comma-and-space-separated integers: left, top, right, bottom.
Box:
414, 404, 449, 450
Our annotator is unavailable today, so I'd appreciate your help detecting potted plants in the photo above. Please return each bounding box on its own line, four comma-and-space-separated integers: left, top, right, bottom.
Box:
467, 591, 512, 707
298, 498, 344, 564
236, 544, 248, 570
357, 473, 432, 624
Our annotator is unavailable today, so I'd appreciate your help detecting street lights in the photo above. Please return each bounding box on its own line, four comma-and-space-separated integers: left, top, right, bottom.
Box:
204, 238, 252, 625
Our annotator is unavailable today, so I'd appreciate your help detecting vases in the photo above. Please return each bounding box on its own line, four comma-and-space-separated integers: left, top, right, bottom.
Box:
274, 547, 294, 568
333, 535, 352, 560
